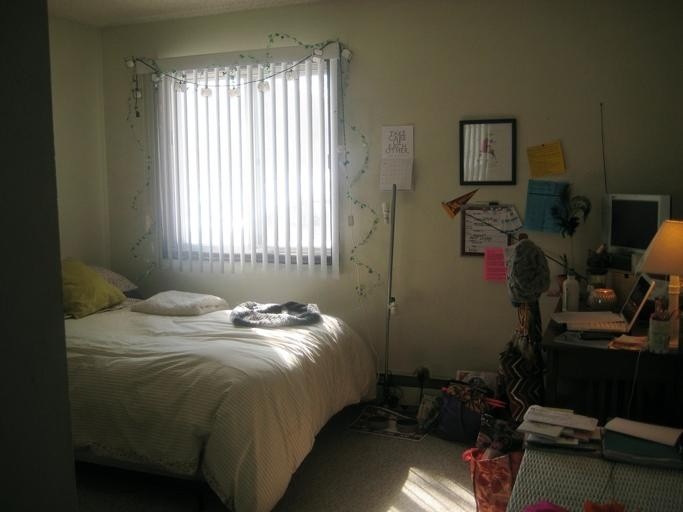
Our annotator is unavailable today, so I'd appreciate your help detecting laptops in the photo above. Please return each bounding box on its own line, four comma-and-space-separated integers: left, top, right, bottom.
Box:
567, 270, 656, 331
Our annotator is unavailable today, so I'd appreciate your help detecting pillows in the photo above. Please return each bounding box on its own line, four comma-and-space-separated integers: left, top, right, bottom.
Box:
58, 258, 141, 321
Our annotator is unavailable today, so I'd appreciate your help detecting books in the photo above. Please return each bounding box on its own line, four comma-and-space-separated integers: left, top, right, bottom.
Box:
515, 405, 683, 468
608, 336, 648, 352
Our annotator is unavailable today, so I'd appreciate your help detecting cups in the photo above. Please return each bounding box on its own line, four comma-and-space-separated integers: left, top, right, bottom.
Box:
647, 311, 672, 354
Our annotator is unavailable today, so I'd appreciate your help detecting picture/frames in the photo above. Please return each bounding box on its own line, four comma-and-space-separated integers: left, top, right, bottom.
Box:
456, 114, 520, 189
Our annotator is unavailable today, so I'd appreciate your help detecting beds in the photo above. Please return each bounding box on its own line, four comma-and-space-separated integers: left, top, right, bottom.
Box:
60, 258, 379, 512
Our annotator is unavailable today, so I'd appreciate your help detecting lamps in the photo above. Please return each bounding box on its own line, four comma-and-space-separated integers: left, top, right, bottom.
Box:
632, 214, 682, 347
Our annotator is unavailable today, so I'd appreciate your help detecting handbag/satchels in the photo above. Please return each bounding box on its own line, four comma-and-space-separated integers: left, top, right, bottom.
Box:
431, 378, 496, 441
468, 447, 524, 512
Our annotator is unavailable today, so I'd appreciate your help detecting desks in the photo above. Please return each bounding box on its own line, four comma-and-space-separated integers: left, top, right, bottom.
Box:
538, 277, 683, 453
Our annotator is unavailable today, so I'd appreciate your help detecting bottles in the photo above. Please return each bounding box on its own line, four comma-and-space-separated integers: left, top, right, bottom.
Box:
561, 269, 580, 312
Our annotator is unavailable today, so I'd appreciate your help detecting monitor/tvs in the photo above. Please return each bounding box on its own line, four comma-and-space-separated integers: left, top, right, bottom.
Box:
605, 193, 672, 256
